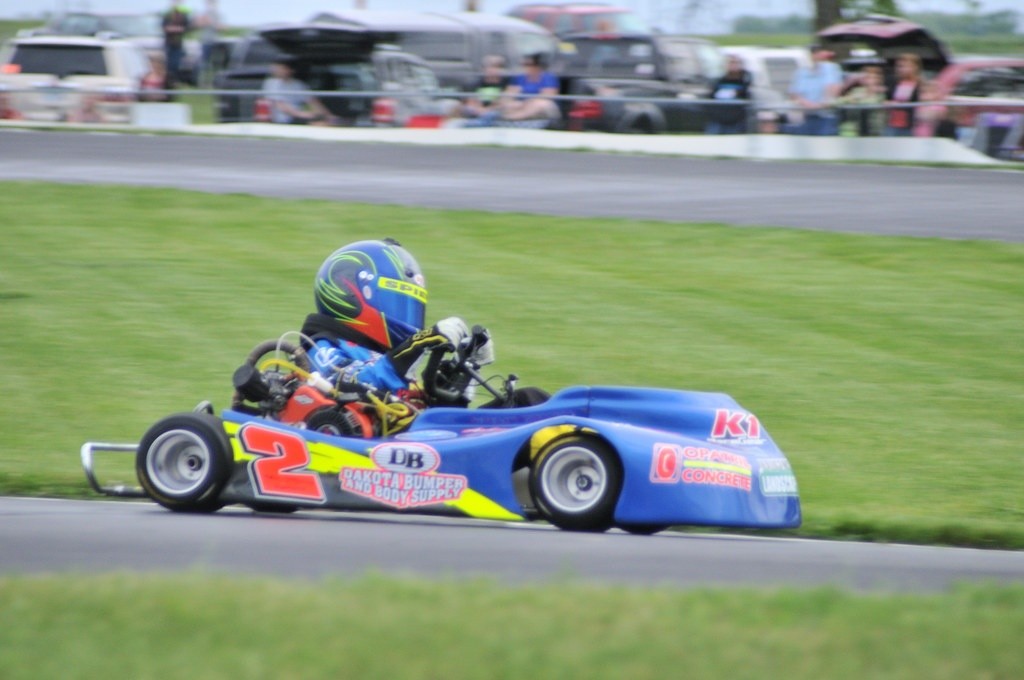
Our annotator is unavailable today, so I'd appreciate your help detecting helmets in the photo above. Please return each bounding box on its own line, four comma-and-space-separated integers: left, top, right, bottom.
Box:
314, 237, 426, 348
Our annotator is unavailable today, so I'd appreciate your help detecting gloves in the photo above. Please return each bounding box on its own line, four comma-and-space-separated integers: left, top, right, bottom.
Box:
457, 329, 494, 403
388, 317, 468, 381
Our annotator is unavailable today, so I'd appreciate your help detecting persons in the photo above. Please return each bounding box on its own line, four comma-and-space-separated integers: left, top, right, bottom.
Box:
140, 0, 563, 130
299, 240, 494, 432
701, 49, 960, 140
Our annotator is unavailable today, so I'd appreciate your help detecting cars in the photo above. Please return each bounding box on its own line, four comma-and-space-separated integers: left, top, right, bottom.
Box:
2, 1, 1023, 137
